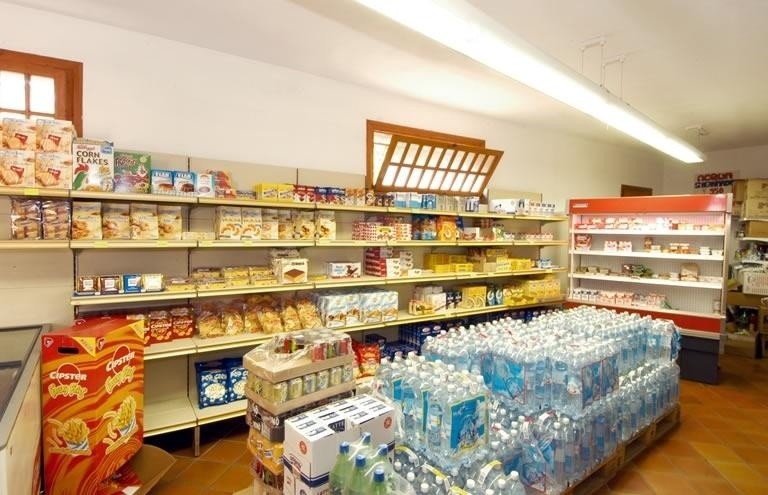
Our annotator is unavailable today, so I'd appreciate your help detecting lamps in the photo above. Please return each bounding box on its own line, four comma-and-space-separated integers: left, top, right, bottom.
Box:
354, 0, 706, 166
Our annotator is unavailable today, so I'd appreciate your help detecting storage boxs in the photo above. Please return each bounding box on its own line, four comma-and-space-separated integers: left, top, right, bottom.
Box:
719, 175, 767, 362
38, 318, 178, 495
282, 393, 396, 495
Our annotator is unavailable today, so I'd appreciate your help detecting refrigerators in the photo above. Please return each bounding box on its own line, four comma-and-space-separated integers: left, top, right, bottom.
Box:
0, 323, 54, 495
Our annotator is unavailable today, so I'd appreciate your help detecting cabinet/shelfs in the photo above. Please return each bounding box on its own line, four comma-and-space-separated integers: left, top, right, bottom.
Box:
0, 146, 570, 459
565, 192, 731, 341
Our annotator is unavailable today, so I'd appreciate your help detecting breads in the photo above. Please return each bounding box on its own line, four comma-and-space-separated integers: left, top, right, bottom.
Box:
159, 184, 173, 191
284, 269, 304, 282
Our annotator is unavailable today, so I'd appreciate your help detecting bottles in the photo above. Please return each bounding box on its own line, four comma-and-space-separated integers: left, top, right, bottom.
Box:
327, 303, 683, 495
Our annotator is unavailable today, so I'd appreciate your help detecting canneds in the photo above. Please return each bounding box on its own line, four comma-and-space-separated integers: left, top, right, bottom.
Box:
247, 326, 356, 490
